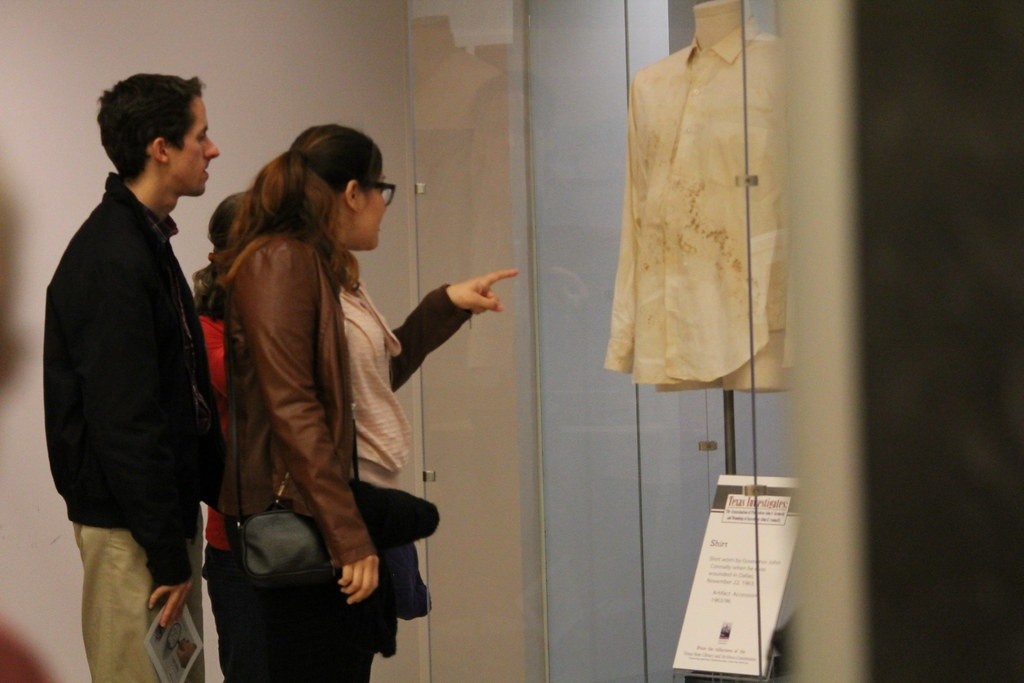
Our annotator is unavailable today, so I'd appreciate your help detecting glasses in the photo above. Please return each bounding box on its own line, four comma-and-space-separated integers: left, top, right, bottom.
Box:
356, 179, 395, 206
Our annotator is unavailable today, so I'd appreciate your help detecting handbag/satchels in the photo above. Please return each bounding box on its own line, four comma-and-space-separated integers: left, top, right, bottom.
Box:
241, 510, 332, 580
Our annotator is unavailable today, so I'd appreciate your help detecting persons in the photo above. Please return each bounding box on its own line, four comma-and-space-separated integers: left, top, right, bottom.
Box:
41, 72, 220, 683
191, 123, 518, 683
